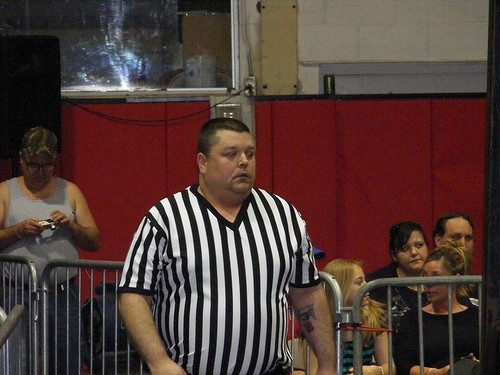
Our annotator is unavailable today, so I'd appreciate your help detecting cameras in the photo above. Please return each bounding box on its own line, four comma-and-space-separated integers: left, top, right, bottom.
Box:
39, 218, 58, 230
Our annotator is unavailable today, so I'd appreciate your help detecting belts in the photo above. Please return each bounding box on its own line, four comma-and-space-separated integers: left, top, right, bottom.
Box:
4, 277, 75, 293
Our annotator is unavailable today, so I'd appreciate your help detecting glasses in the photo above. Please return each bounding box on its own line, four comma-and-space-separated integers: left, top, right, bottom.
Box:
28, 163, 55, 170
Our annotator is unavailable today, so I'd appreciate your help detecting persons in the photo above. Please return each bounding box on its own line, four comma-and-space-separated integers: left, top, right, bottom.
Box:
300, 257, 397, 375
115, 117, 339, 375
365, 221, 433, 325
0, 126, 101, 375
391, 240, 481, 375
431, 211, 480, 307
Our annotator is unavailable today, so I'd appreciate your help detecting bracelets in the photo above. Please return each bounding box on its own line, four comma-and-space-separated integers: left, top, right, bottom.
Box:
14, 222, 24, 240
428, 368, 435, 375
378, 365, 385, 375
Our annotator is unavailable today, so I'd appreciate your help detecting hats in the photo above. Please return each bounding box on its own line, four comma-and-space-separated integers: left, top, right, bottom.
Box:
22, 127, 58, 166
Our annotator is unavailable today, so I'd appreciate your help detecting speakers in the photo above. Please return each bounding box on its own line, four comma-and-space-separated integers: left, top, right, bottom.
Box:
0, 34, 62, 161
